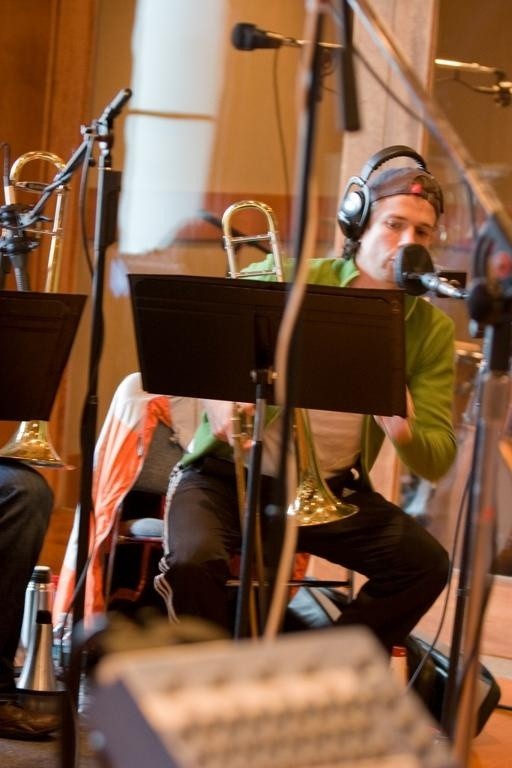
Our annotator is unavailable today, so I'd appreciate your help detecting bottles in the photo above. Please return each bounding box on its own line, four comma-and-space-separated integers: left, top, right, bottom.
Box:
19, 562, 56, 650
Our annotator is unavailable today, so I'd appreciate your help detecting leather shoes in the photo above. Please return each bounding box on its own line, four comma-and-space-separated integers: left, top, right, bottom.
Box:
0, 699, 65, 740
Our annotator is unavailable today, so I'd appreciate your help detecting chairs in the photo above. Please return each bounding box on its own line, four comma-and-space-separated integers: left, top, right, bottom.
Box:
214, 502, 356, 639
71, 373, 212, 673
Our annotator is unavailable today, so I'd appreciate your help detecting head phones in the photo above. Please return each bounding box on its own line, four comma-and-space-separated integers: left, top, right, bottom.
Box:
336, 144, 428, 242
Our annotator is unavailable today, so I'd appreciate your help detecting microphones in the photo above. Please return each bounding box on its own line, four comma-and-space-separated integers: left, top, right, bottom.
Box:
419, 273, 464, 298
230, 22, 296, 51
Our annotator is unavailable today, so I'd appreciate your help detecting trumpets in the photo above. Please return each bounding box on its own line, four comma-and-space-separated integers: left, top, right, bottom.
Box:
221, 199, 360, 639
1, 150, 75, 469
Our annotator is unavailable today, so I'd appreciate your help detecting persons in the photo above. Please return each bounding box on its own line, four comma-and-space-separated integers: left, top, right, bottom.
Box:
0, 252, 64, 742
154, 166, 460, 662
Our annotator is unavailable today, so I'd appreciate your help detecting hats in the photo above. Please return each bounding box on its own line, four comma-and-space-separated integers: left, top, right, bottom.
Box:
364, 166, 443, 216
